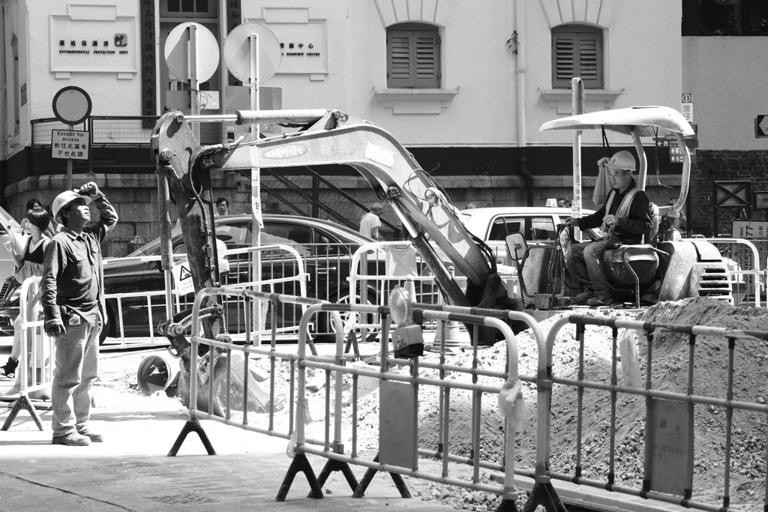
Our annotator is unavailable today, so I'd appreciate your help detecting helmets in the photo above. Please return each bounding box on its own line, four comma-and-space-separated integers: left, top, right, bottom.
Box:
51, 189, 93, 225
603, 150, 637, 172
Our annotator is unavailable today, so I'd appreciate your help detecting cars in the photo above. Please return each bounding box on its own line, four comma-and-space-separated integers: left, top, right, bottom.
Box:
100, 211, 455, 350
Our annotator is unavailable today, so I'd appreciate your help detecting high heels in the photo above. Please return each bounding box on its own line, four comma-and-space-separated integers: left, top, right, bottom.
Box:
0, 356, 19, 378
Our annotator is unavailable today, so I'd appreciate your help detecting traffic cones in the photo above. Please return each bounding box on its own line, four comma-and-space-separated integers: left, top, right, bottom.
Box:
398, 280, 416, 326
433, 265, 463, 343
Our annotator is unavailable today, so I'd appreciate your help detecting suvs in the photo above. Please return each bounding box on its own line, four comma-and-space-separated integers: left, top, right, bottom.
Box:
438, 198, 746, 304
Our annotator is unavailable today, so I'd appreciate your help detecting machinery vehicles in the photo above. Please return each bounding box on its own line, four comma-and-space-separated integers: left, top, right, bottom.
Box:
150, 106, 735, 415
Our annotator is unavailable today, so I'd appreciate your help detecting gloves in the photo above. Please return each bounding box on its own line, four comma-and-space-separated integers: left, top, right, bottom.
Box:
43, 304, 68, 337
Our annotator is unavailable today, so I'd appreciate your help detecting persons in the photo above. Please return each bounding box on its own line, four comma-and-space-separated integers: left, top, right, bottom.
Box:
20, 197, 55, 240
213, 198, 230, 217
557, 197, 571, 207
0, 208, 51, 379
38, 180, 119, 445
564, 150, 651, 307
358, 202, 384, 240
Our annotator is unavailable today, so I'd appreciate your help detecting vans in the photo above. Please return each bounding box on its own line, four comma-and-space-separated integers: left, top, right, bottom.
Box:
0, 206, 31, 334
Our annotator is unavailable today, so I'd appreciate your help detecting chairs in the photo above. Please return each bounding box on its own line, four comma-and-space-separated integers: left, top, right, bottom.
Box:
557, 203, 660, 296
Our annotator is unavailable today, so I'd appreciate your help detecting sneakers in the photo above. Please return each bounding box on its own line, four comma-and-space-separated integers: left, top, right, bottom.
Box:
575, 289, 615, 306
52, 429, 104, 447
